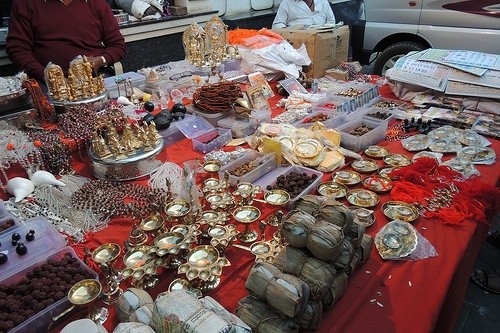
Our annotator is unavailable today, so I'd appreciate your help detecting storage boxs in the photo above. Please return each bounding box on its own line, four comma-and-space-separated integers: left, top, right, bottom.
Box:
0, 25, 392, 333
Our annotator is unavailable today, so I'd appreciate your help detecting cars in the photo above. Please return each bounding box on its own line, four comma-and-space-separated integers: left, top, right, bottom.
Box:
361, 0, 500, 78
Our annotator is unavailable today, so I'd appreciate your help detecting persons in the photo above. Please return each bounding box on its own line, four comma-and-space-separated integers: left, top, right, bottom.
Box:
67, 69, 80, 94
272, 0, 336, 33
212, 30, 220, 51
82, 54, 93, 80
65, 76, 105, 101
191, 34, 199, 63
48, 72, 58, 100
5, 0, 127, 82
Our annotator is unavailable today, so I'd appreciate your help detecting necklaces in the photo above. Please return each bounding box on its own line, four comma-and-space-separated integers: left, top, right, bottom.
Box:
21, 78, 57, 129
60, 103, 107, 162
30, 128, 75, 177
0, 128, 43, 191
72, 180, 170, 218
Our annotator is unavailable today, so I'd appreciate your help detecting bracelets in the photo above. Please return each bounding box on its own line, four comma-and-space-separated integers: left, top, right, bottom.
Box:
337, 171, 352, 182
356, 190, 374, 205
327, 182, 341, 195
355, 145, 404, 190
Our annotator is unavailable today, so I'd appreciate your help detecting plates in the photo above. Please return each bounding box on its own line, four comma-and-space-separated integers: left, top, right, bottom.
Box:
317, 125, 496, 261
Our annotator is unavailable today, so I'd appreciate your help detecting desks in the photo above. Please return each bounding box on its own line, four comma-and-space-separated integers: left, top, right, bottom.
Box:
0, 73, 500, 333
